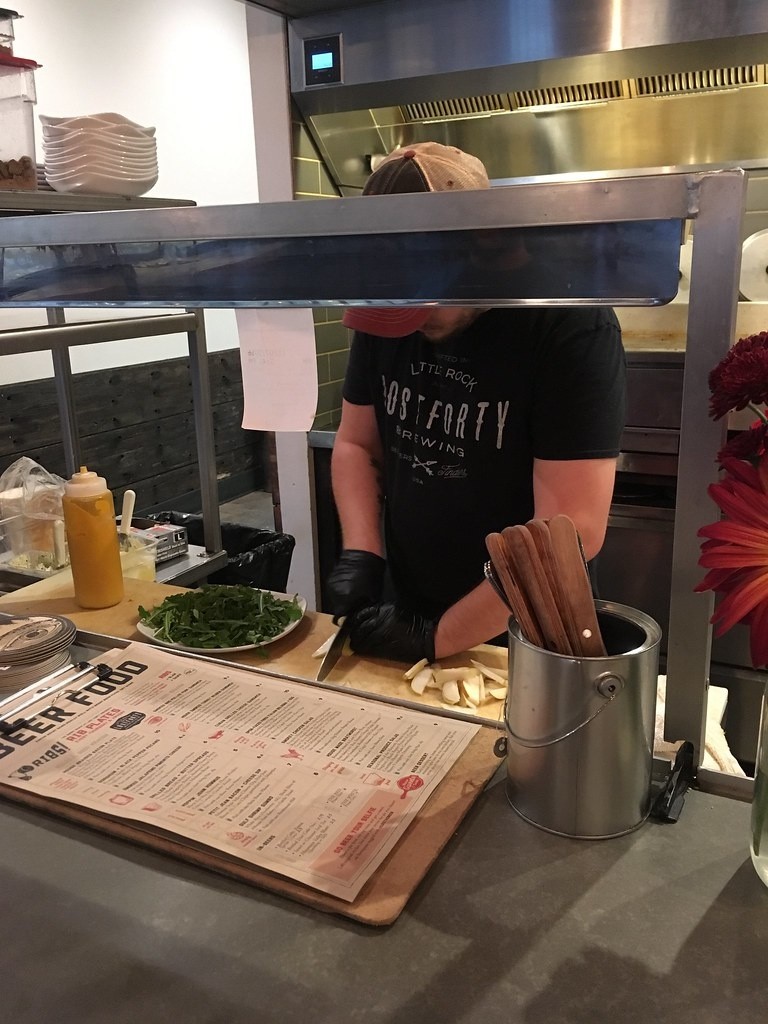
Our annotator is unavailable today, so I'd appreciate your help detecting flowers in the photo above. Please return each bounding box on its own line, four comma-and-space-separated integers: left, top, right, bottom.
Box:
691, 331, 768, 858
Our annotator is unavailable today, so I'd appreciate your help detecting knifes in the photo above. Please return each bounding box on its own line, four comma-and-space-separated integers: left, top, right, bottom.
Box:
316, 614, 351, 682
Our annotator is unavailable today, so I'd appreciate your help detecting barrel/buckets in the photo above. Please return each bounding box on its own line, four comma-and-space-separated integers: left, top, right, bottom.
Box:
504, 598, 662, 841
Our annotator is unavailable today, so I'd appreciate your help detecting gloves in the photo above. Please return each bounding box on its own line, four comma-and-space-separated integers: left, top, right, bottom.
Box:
321, 549, 387, 626
348, 597, 443, 666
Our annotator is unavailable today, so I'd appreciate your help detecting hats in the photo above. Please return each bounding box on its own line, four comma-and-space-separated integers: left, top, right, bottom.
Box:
342, 140, 490, 338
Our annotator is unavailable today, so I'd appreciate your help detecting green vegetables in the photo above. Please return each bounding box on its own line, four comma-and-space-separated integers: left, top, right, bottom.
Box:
137, 583, 302, 659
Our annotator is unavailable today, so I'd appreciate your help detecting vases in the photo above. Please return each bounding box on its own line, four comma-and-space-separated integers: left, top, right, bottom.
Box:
748, 677, 768, 888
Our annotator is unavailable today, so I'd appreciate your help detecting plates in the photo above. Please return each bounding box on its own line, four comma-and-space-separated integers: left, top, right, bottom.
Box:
137, 587, 307, 653
37, 112, 158, 195
0, 614, 77, 694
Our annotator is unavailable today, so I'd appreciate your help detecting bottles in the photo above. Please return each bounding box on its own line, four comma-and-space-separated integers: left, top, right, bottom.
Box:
750, 681, 768, 888
61, 465, 125, 610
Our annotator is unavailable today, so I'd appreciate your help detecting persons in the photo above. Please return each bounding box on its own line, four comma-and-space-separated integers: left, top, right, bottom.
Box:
330, 143, 628, 663
